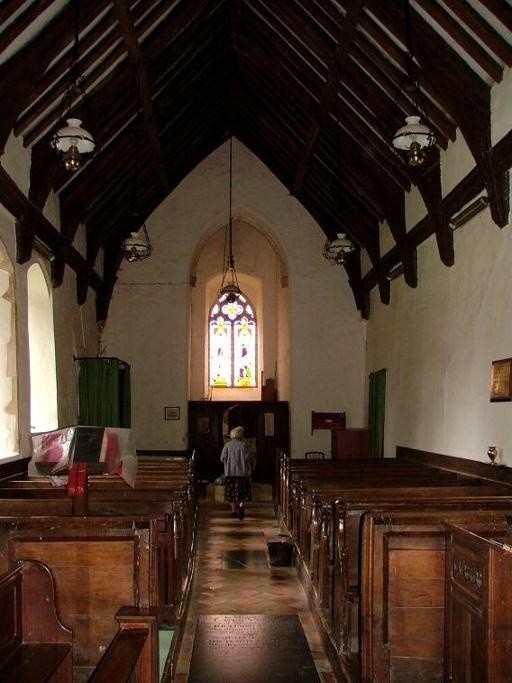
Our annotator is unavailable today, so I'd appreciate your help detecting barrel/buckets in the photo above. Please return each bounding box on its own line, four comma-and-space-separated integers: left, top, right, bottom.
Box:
267, 534, 295, 567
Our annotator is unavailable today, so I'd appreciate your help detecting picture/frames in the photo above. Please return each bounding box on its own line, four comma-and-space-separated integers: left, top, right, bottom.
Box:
490, 358, 512, 402
165, 407, 180, 420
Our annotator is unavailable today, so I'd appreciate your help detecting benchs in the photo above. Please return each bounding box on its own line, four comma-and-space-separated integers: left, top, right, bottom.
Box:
1, 449, 200, 682
274, 448, 512, 682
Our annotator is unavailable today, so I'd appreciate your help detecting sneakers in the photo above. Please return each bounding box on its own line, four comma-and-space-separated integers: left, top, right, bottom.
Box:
231, 508, 245, 520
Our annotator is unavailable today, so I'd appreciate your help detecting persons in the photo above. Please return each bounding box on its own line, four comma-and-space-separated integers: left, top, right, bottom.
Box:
221, 426, 256, 519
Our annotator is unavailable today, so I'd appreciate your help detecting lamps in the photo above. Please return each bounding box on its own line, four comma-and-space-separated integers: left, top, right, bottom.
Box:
322, 0, 439, 265
218, 1, 243, 304
49, 0, 156, 265
